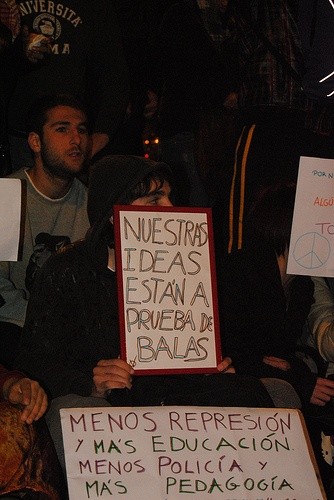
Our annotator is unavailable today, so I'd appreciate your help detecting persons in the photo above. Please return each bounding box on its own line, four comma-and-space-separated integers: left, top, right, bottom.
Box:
0, 96, 89, 370
0, 359, 63, 500
213, 180, 334, 408
0, 0, 333, 186
288, 275, 334, 500
17, 153, 277, 472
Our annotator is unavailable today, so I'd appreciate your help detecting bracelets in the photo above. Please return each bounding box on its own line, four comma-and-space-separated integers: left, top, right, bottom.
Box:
2, 377, 20, 401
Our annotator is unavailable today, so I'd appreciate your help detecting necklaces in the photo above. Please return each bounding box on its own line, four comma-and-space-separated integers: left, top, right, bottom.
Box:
24, 185, 65, 255
